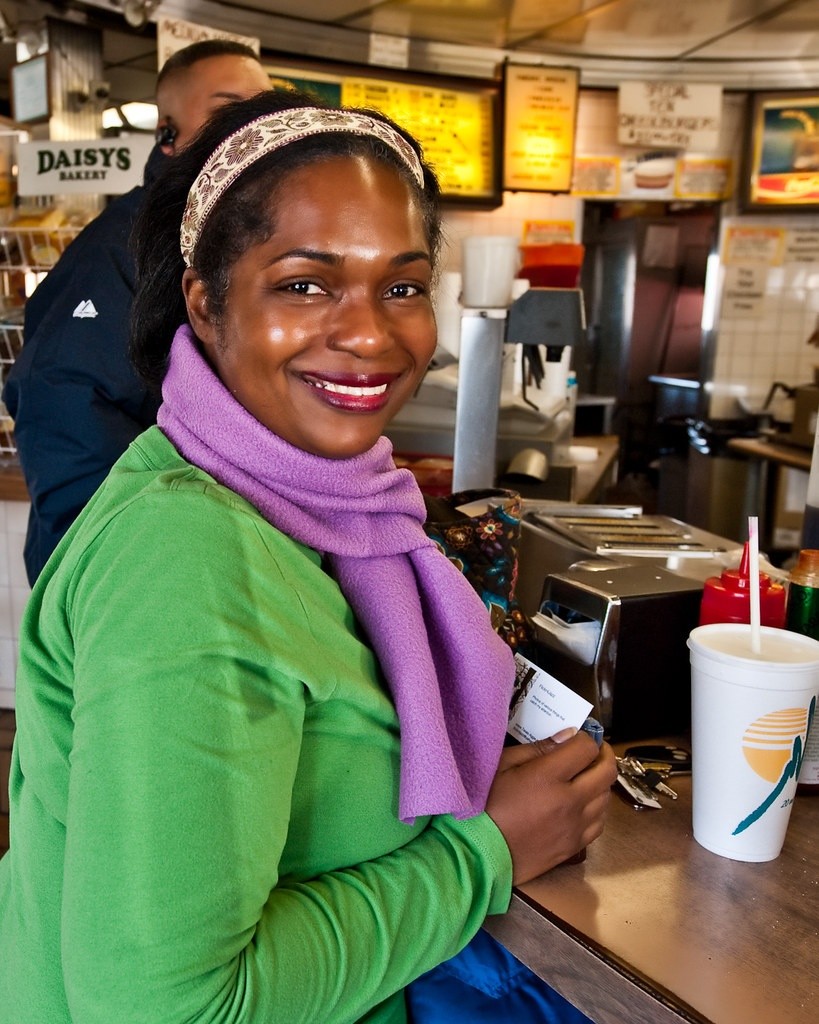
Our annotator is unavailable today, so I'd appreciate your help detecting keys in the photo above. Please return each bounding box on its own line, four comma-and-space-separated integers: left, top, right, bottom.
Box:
612, 744, 691, 808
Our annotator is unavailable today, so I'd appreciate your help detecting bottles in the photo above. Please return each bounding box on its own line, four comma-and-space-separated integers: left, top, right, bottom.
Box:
785, 549, 819, 796
700, 539, 785, 628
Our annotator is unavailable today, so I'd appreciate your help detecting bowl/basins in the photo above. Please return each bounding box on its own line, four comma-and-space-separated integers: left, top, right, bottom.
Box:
634, 172, 672, 190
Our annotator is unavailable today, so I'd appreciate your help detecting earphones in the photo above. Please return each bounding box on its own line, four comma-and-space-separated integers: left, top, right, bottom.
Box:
155, 127, 177, 146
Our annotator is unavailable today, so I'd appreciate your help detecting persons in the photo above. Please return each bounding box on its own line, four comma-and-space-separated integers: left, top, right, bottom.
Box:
2, 39, 273, 591
0, 88, 618, 1024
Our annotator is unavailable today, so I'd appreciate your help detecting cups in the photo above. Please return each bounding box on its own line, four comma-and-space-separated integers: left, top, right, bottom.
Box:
686, 623, 819, 861
791, 127, 819, 172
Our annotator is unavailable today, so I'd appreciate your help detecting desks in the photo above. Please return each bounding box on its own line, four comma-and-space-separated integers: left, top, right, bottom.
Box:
723, 436, 813, 551
461, 723, 819, 1023
